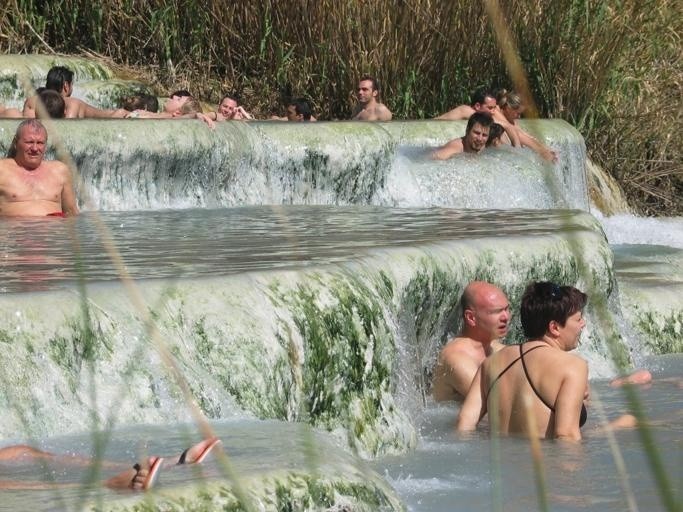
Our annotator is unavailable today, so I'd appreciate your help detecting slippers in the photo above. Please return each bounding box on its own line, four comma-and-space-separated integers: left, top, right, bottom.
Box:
176, 437, 221, 467
126, 455, 165, 491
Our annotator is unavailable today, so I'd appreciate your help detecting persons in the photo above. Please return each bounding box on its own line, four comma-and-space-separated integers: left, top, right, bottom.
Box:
457, 280, 639, 442
433, 278, 653, 404
0, 119, 80, 219
350, 76, 392, 121
432, 111, 492, 160
270, 98, 316, 122
0, 434, 223, 494
1, 65, 254, 123
429, 86, 520, 150
493, 83, 558, 166
485, 123, 508, 150
